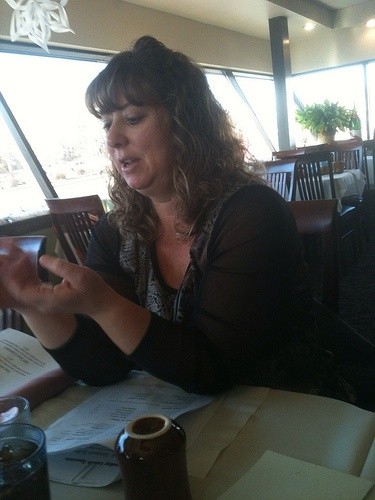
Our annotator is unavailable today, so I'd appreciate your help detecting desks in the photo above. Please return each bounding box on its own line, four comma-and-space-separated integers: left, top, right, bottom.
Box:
268, 155, 375, 185
262, 168, 367, 202
29, 369, 375, 500
55, 228, 93, 267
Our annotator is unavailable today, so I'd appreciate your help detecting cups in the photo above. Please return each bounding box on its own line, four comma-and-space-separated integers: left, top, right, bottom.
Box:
332, 161, 346, 173
0, 424, 51, 500
0, 396, 32, 424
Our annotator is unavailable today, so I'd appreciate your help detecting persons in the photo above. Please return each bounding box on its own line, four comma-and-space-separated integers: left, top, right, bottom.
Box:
0, 36, 334, 406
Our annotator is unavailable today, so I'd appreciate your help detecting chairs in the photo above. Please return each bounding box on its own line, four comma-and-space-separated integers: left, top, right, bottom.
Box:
0, 140, 375, 413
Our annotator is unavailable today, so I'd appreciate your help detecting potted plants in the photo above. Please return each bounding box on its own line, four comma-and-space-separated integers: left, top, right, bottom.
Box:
295, 100, 356, 143
